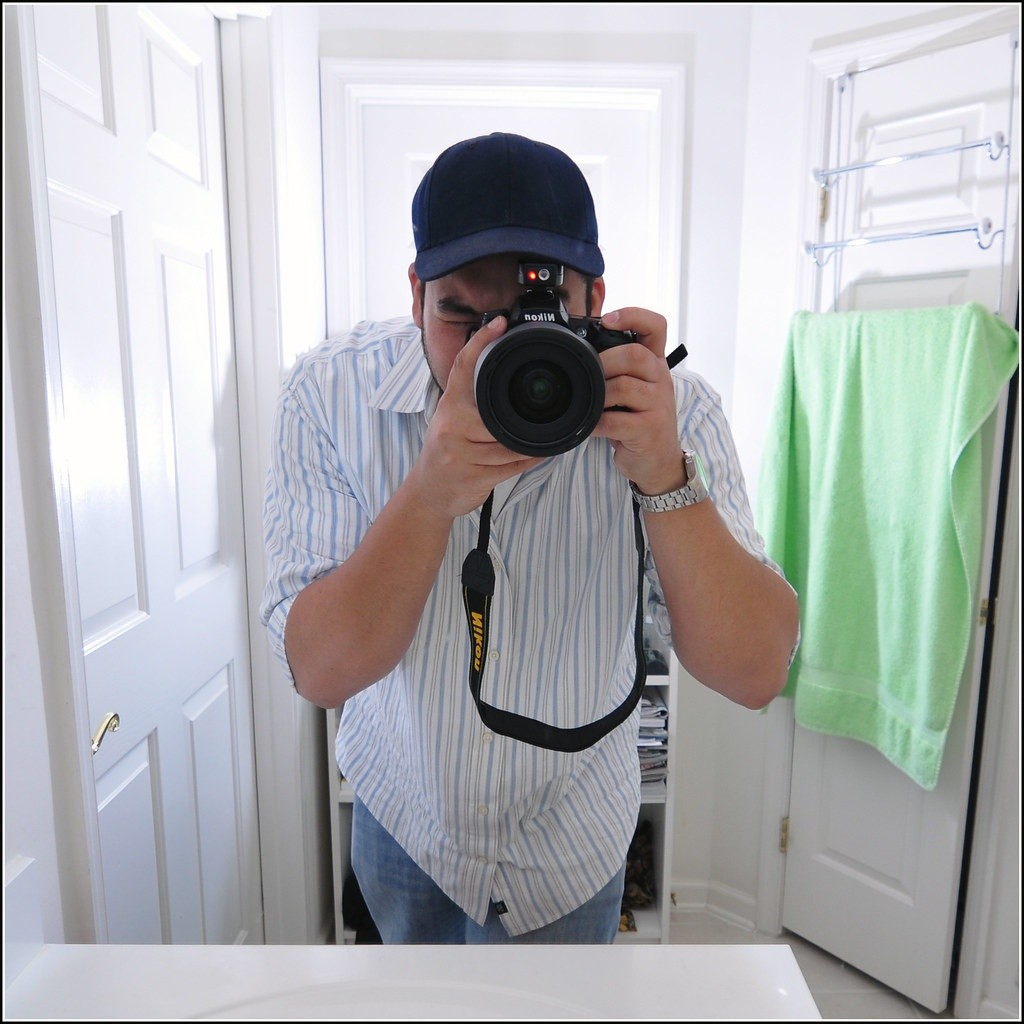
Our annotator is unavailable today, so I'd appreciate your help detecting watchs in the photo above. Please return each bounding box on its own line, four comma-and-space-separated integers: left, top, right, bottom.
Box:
625, 449, 714, 513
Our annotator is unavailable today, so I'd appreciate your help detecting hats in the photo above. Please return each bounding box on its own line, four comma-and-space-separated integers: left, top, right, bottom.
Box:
411, 134, 606, 280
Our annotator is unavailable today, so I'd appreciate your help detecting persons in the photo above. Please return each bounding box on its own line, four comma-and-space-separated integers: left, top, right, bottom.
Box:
259, 128, 806, 945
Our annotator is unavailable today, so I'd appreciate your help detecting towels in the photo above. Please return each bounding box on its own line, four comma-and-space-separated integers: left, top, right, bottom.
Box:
761, 298, 1021, 793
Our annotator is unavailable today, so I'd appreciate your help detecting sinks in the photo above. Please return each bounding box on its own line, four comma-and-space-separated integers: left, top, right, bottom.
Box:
193, 971, 578, 1021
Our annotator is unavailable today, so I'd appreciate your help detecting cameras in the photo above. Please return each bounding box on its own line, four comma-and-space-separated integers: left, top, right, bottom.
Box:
465, 258, 640, 458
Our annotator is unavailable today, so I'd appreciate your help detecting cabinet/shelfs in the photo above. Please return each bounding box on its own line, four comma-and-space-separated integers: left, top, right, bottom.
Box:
323, 620, 680, 949
